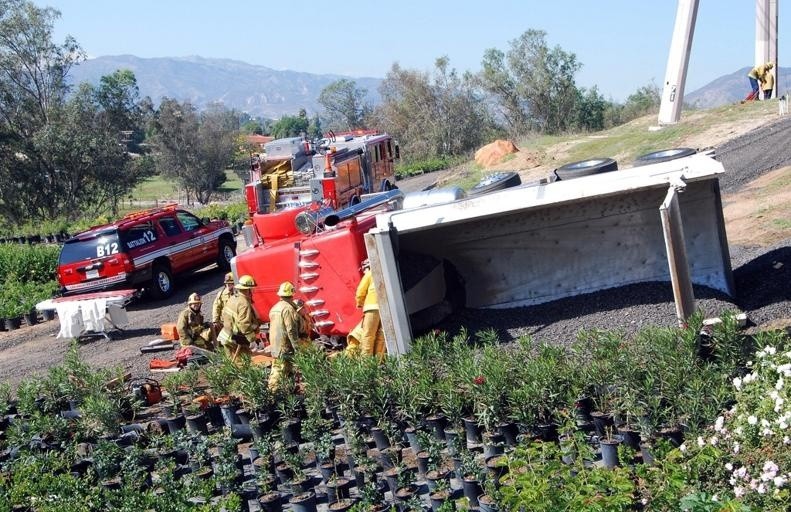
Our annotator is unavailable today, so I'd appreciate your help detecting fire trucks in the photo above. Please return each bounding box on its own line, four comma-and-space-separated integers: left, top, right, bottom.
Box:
242, 127, 402, 217
51, 204, 236, 298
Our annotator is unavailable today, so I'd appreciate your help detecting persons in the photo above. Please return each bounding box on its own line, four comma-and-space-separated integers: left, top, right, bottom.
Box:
745, 62, 774, 101
761, 69, 775, 101
176, 270, 311, 397
354, 257, 389, 358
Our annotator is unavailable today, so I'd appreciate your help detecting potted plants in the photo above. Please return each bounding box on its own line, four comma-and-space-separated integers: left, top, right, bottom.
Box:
0, 220, 115, 330
0, 311, 791, 512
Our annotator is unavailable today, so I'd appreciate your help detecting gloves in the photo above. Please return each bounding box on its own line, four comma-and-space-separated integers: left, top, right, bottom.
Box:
249, 341, 257, 349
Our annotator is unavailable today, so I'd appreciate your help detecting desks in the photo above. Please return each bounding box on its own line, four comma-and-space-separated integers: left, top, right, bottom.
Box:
34, 291, 134, 343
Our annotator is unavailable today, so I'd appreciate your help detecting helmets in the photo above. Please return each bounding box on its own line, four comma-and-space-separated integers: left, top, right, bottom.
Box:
359, 258, 370, 271
234, 275, 258, 289
223, 272, 234, 284
187, 293, 204, 305
276, 281, 296, 297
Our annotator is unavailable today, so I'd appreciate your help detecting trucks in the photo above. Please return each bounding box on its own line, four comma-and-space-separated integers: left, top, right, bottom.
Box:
230, 148, 749, 367
